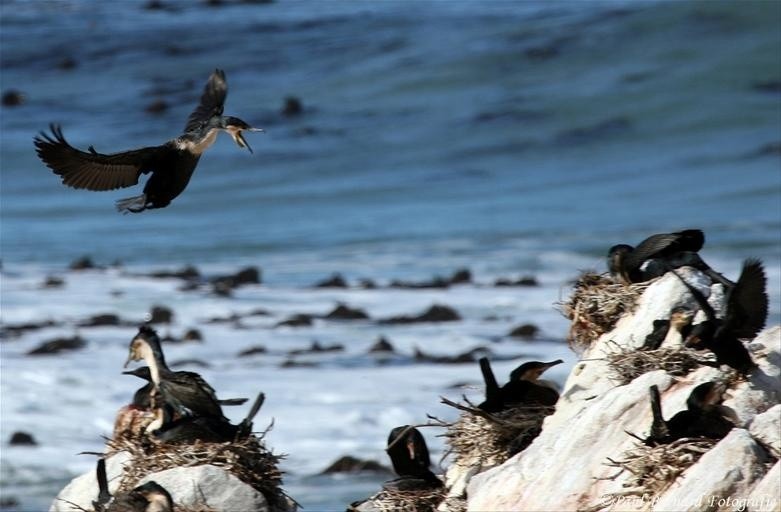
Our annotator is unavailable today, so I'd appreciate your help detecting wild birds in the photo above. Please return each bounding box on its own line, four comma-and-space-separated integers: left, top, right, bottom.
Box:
645, 380, 740, 447
388, 424, 443, 484
33, 67, 264, 216
606, 228, 705, 283
124, 325, 267, 449
634, 305, 698, 352
476, 357, 564, 412
92, 458, 173, 512
667, 255, 770, 381
